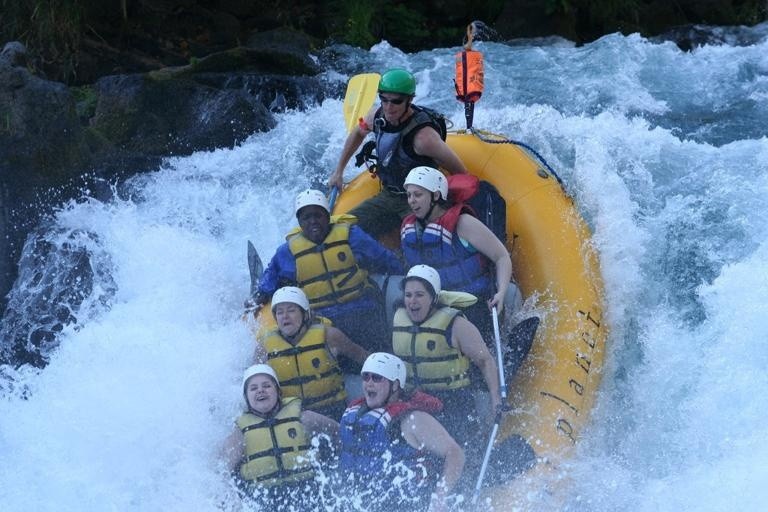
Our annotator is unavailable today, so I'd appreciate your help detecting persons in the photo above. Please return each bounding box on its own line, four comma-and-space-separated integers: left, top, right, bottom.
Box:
338, 351, 465, 511
244, 288, 376, 463
320, 69, 469, 240
234, 187, 409, 372
388, 264, 504, 470
212, 364, 347, 512
395, 167, 514, 395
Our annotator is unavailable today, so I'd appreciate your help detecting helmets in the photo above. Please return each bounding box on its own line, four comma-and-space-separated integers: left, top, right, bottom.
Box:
403, 166, 448, 201
295, 189, 330, 215
361, 351, 406, 389
271, 286, 310, 312
242, 363, 280, 393
378, 69, 416, 95
406, 264, 441, 295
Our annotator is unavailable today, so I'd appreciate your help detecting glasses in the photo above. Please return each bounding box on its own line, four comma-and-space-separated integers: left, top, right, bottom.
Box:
379, 95, 403, 104
362, 372, 383, 382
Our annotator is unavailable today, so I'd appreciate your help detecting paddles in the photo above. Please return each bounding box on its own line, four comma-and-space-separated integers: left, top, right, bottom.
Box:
470, 316, 540, 512
242, 240, 264, 322
328, 73, 381, 216
479, 187, 509, 411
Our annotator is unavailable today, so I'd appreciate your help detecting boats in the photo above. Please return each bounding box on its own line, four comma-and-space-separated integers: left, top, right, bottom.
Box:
221, 130, 608, 510
